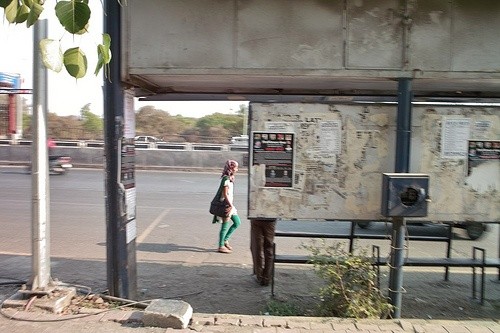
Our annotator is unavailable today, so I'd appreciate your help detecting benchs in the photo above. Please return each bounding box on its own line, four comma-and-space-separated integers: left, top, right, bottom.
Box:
271, 242, 500, 305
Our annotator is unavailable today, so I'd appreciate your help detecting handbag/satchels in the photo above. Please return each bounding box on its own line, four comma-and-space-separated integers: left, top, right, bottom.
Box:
210, 195, 230, 218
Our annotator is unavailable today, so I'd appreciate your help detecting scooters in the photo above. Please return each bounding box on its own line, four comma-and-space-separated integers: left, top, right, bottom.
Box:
28, 155, 71, 175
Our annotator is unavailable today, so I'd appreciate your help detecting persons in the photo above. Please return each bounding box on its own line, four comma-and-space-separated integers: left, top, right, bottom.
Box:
250, 218, 278, 287
211, 161, 241, 254
47, 136, 56, 155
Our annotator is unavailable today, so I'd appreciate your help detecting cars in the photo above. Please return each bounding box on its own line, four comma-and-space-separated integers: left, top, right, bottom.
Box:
135, 135, 161, 143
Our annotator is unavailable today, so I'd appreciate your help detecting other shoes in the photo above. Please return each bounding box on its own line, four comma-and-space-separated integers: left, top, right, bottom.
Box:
218, 245, 231, 254
224, 242, 233, 251
